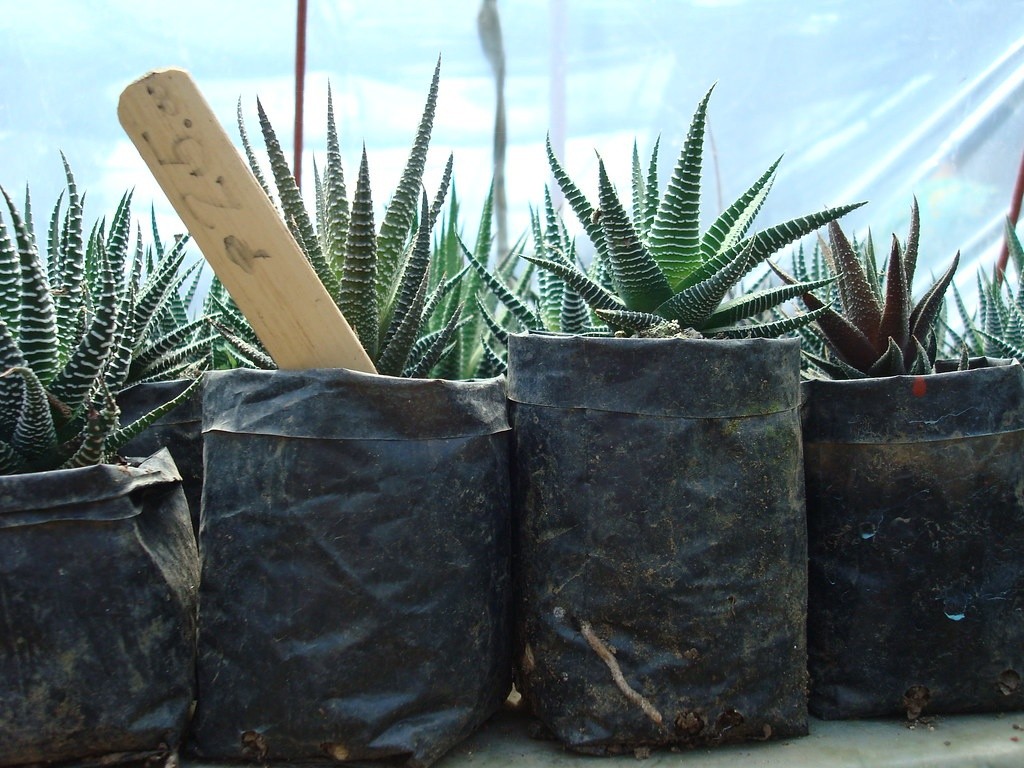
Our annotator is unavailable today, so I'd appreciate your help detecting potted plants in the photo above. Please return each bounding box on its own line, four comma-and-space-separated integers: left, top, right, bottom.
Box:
0, 86, 1024, 768
